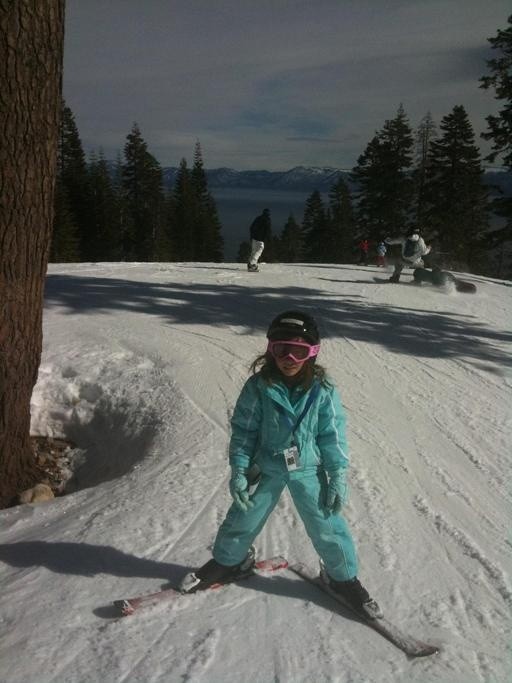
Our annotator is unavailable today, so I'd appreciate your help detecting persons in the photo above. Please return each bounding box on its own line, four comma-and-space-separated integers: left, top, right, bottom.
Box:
177, 310, 371, 604
356, 238, 369, 266
375, 240, 387, 267
246, 208, 273, 272
383, 225, 432, 285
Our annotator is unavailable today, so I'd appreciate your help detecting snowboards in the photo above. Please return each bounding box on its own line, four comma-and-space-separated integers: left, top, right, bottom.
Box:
414, 267, 476, 292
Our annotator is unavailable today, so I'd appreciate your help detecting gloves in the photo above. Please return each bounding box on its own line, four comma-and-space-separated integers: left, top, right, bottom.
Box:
231, 470, 254, 512
326, 468, 347, 516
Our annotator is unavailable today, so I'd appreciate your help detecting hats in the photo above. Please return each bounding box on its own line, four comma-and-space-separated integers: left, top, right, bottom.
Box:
409, 225, 419, 233
263, 209, 271, 216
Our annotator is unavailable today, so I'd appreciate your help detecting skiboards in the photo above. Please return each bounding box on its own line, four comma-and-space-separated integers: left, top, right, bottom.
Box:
115, 556, 441, 658
374, 277, 414, 285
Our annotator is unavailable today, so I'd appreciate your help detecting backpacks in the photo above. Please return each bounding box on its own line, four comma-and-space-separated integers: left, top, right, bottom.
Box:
404, 236, 420, 257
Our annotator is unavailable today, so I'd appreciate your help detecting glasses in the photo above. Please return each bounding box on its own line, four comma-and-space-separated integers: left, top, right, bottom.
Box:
270, 341, 312, 363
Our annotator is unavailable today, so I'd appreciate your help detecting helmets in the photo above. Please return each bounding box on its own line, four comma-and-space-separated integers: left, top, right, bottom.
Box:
267, 311, 319, 345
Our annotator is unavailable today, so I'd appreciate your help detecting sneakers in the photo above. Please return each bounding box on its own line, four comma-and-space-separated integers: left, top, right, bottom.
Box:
196, 559, 248, 583
326, 572, 369, 603
248, 263, 258, 270
